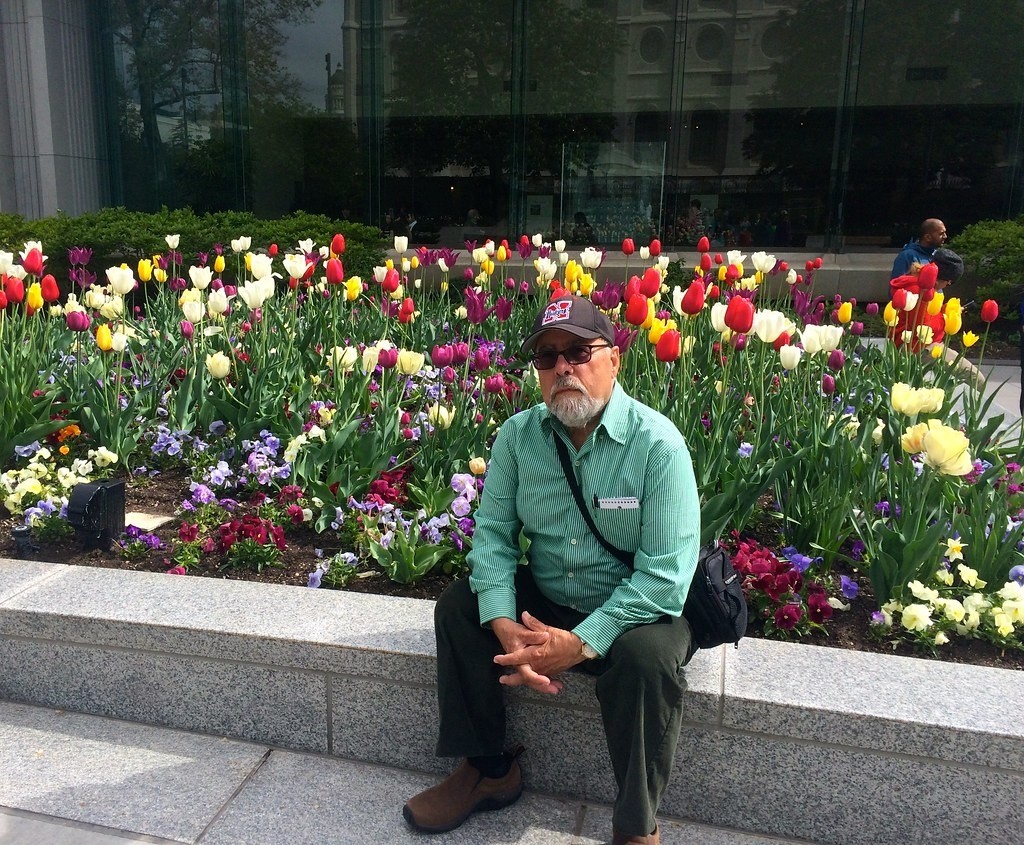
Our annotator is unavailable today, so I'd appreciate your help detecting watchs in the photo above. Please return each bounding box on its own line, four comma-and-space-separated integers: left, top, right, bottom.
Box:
579, 638, 600, 660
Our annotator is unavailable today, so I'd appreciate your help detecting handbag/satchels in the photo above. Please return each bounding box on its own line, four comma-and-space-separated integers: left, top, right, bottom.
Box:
683, 546, 748, 649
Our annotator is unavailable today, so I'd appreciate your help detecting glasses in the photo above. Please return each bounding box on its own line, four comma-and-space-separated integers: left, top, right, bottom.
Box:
529, 344, 612, 370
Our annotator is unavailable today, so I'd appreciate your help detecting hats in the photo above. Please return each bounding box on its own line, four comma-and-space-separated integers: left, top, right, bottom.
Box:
520, 296, 615, 355
930, 249, 964, 283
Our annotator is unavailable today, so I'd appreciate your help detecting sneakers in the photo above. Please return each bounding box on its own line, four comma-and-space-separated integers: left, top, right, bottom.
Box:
612, 819, 659, 845
403, 745, 525, 831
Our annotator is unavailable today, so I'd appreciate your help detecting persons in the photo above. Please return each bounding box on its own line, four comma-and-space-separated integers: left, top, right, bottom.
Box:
712, 208, 809, 248
571, 212, 598, 245
403, 296, 701, 845
889, 218, 947, 294
340, 208, 416, 243
464, 209, 486, 242
888, 248, 985, 392
677, 199, 707, 236
